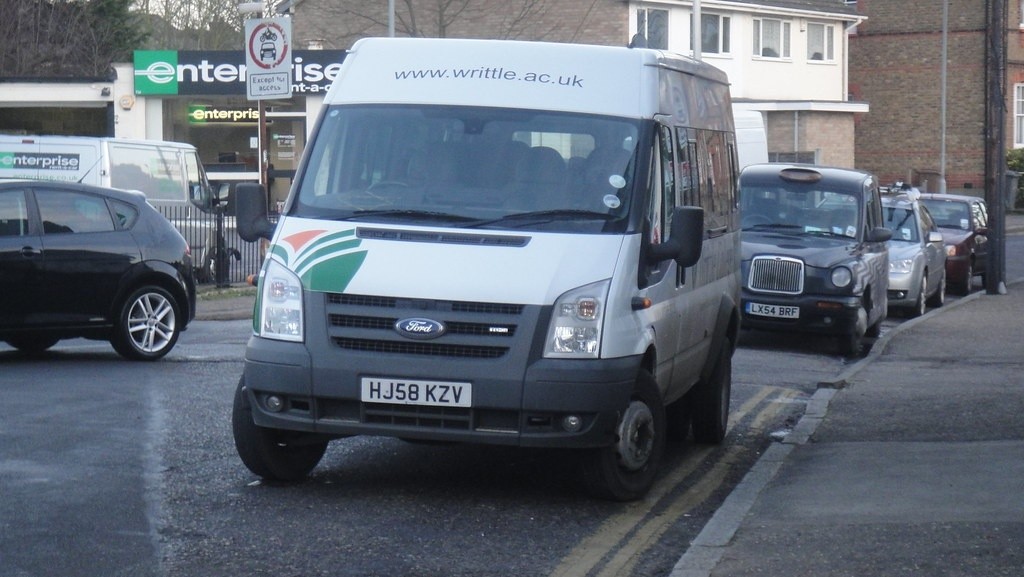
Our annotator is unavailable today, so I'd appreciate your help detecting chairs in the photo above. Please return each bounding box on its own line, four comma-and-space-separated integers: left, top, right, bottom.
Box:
948, 210, 965, 226
828, 209, 857, 235
745, 197, 779, 225
409, 139, 632, 207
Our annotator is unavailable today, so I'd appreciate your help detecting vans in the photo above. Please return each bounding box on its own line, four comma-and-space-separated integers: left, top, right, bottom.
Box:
231, 36, 746, 504
0, 131, 227, 283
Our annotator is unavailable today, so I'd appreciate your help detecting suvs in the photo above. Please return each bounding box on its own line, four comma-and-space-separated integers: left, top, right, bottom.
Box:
1, 177, 198, 363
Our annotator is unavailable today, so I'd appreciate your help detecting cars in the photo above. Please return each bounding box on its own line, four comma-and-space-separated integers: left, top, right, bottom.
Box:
878, 185, 947, 317
735, 158, 891, 354
920, 191, 986, 301
